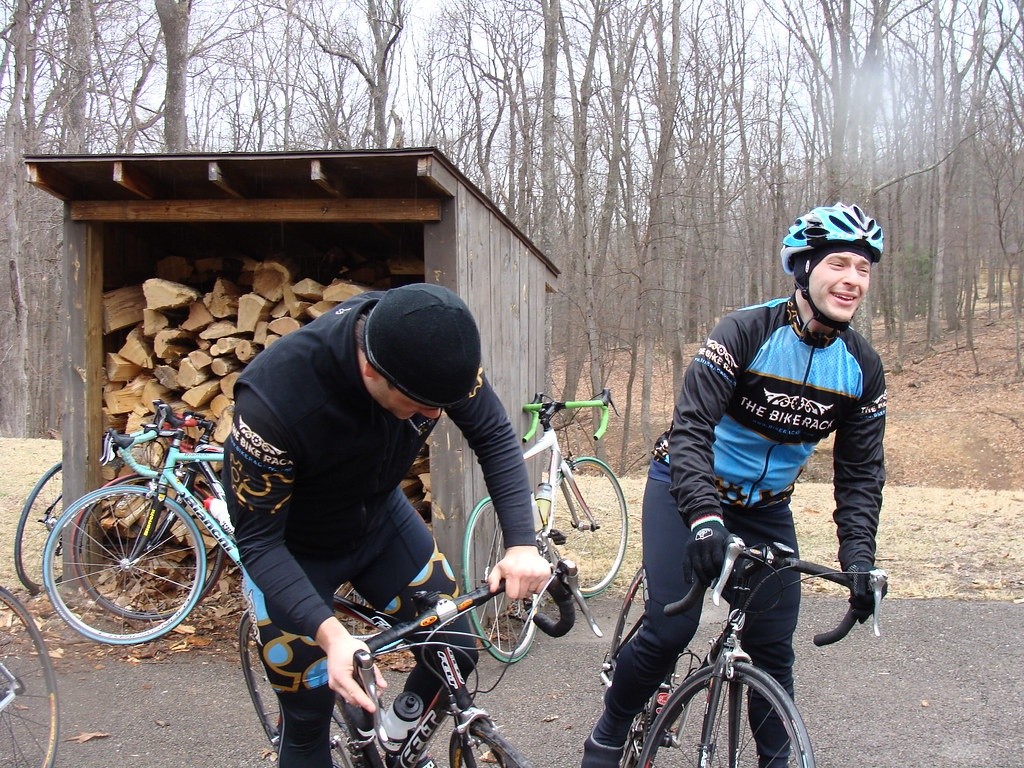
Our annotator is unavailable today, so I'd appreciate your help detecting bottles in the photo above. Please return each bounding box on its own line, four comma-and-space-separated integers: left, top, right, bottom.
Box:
536, 483, 552, 528
381, 691, 424, 753
202, 496, 235, 535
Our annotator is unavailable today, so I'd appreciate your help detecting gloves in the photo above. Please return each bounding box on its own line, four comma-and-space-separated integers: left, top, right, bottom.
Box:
845, 561, 887, 624
683, 511, 731, 587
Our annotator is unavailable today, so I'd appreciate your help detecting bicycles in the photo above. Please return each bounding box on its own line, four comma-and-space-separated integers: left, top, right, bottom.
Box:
41, 398, 387, 646
14, 462, 62, 592
461, 386, 629, 664
0, 587, 60, 768
598, 530, 888, 768
239, 556, 604, 768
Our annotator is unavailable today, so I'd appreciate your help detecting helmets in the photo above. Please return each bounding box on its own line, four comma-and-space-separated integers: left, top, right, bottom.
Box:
781, 202, 884, 275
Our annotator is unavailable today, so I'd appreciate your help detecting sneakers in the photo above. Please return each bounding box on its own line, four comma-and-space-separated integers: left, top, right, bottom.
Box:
386, 753, 438, 768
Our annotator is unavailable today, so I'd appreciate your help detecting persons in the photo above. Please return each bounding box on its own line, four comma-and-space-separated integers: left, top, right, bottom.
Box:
581, 202, 887, 768
223, 283, 551, 768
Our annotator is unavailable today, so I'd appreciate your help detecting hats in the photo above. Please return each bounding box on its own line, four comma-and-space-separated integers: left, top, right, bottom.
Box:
364, 283, 481, 407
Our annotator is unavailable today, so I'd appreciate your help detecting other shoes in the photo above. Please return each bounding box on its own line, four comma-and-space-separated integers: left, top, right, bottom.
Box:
581, 725, 624, 768
